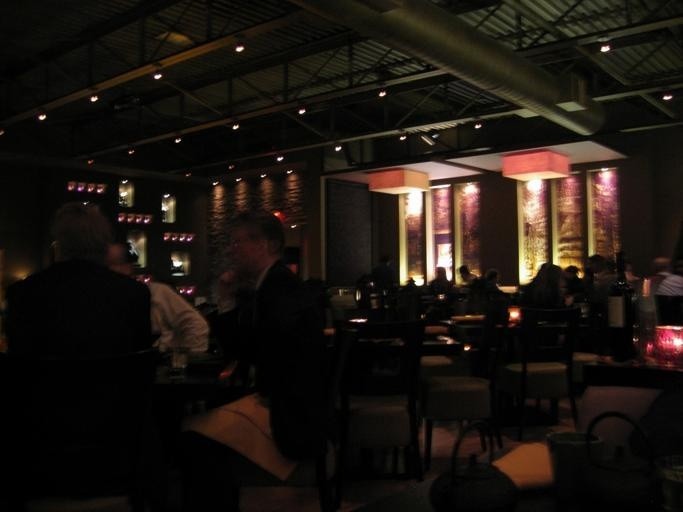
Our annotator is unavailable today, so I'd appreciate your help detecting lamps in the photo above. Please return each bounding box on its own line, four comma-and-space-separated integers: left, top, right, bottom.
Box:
0, 36, 676, 199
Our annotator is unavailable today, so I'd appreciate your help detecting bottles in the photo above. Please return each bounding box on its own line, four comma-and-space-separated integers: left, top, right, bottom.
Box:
607, 252, 633, 362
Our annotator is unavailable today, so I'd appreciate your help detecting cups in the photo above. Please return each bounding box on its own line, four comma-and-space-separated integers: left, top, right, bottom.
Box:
546, 431, 603, 491
167, 342, 191, 373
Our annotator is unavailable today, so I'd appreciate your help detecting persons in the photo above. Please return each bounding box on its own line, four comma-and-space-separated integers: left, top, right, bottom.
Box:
0, 201, 682, 510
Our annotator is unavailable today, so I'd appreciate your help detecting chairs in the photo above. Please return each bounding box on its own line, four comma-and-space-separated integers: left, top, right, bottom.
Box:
1, 277, 683, 511
